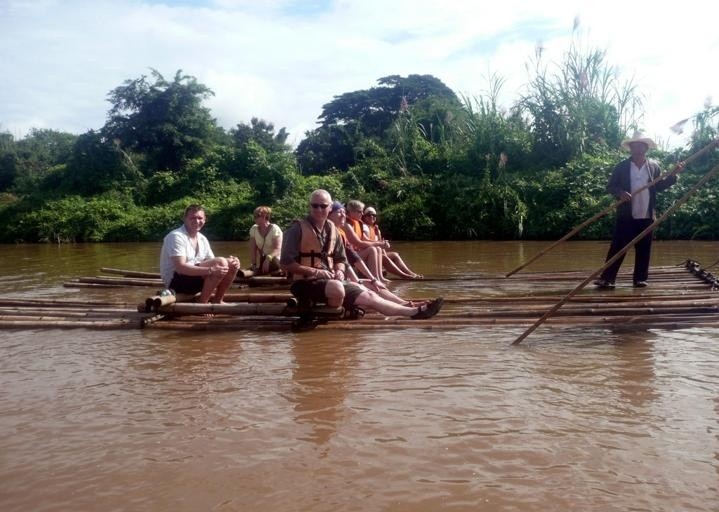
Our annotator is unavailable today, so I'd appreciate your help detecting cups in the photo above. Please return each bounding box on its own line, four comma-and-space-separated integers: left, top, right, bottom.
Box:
160, 288, 176, 297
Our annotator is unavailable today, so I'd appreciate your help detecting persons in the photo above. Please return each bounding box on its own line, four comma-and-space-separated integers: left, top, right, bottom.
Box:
328, 200, 433, 307
362, 206, 423, 279
595, 131, 685, 290
341, 200, 392, 282
279, 188, 444, 320
248, 205, 283, 274
161, 204, 240, 317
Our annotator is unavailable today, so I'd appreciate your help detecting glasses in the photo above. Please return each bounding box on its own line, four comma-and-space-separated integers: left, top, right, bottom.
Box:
367, 214, 377, 217
310, 203, 329, 210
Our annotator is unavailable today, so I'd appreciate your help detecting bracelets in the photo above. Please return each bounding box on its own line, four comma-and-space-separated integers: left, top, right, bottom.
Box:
209, 266, 214, 275
371, 278, 377, 284
265, 254, 272, 261
251, 263, 257, 266
315, 268, 321, 277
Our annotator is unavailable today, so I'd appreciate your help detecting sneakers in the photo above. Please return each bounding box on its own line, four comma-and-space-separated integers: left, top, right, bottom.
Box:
410, 295, 444, 320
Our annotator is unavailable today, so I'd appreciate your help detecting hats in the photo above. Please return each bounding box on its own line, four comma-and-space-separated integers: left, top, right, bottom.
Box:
620, 131, 657, 151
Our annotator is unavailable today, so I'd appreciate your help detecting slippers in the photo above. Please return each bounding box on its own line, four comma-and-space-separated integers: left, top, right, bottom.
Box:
593, 279, 616, 288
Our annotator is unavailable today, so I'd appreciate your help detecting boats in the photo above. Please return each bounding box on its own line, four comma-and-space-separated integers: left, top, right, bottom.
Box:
56, 257, 716, 290
0, 285, 719, 333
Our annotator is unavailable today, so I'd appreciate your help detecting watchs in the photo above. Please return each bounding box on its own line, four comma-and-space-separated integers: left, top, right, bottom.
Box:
356, 280, 364, 285
